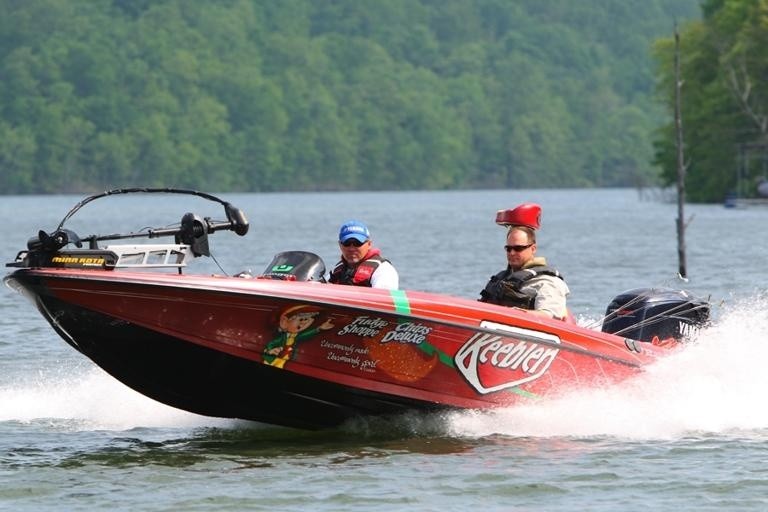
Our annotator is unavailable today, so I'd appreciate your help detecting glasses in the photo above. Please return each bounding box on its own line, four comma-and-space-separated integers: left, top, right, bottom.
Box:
343, 240, 364, 247
504, 244, 533, 252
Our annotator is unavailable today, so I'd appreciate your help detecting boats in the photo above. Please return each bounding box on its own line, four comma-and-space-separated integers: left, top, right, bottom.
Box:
725, 197, 767, 208
0, 188, 713, 434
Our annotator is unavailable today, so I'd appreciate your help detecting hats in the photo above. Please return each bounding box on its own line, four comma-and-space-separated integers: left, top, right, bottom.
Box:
339, 220, 370, 242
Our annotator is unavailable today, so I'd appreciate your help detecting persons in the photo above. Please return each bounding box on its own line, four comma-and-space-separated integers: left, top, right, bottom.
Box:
477, 226, 570, 321
325, 221, 399, 290
